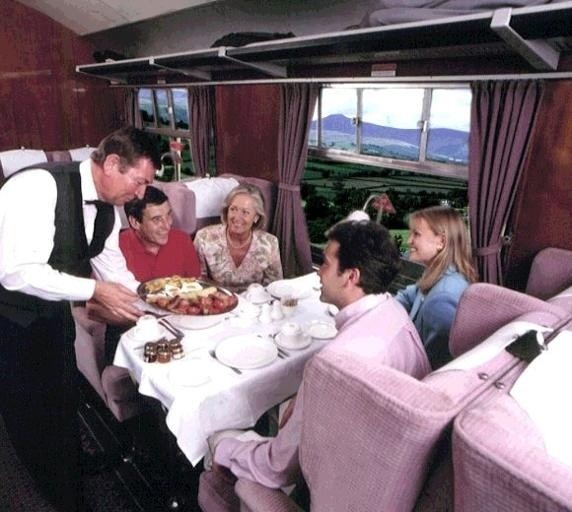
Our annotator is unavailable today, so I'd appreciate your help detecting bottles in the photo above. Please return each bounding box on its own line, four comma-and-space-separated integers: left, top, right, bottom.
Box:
143, 343, 158, 363
157, 341, 170, 363
270, 300, 283, 321
258, 302, 272, 324
169, 339, 185, 360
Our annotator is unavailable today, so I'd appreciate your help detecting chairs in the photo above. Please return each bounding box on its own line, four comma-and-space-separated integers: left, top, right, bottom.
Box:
197, 283, 559, 511
1, 147, 53, 180
452, 322, 571, 512
71, 184, 194, 423
170, 173, 273, 239
56, 146, 101, 168
449, 248, 571, 356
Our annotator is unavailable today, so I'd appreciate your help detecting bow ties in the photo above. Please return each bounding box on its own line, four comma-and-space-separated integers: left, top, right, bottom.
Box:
85, 200, 104, 210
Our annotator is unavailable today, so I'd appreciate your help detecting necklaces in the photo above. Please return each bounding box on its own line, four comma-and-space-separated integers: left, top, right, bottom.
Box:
227, 226, 252, 249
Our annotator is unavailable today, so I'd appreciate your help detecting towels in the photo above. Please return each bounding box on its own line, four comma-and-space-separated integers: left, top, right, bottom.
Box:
279, 283, 296, 295
241, 345, 258, 366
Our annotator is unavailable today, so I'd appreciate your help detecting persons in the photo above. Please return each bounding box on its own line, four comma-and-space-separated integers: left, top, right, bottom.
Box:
0, 126, 161, 512
85, 186, 202, 365
203, 220, 432, 496
393, 206, 477, 366
193, 183, 283, 292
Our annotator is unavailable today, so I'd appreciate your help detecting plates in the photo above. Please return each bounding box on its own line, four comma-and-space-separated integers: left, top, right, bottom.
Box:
304, 319, 337, 339
240, 290, 272, 305
216, 334, 279, 369
265, 279, 313, 299
274, 331, 313, 350
137, 276, 239, 331
128, 324, 165, 345
327, 304, 339, 316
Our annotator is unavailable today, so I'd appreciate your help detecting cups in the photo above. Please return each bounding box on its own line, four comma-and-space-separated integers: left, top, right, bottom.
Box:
134, 315, 159, 340
279, 321, 303, 346
246, 282, 266, 302
281, 305, 299, 318
